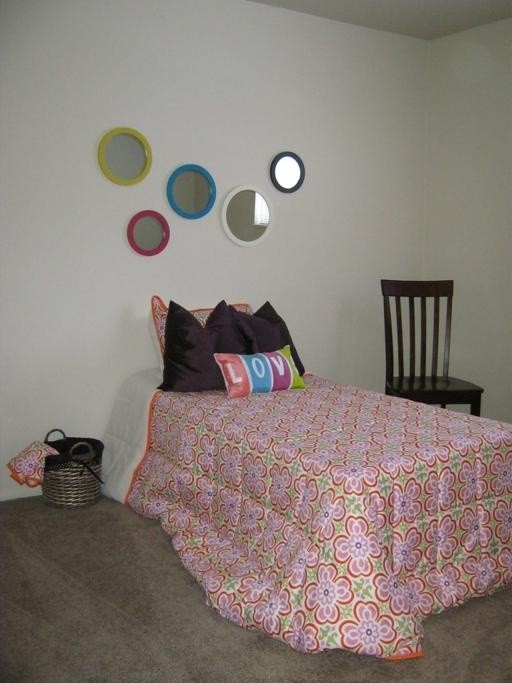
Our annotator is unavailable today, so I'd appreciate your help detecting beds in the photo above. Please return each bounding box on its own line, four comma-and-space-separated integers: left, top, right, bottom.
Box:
96, 296, 512, 662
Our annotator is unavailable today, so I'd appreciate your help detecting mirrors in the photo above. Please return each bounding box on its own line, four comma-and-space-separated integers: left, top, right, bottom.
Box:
269, 151, 306, 193
126, 210, 170, 256
166, 164, 217, 220
97, 128, 152, 186
221, 185, 273, 247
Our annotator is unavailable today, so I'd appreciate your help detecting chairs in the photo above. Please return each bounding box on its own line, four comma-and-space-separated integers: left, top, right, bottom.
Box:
380, 279, 484, 417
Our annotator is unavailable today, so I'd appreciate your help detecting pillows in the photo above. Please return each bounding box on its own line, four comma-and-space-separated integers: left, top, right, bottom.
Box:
156, 299, 251, 392
213, 345, 306, 399
151, 295, 253, 360
227, 301, 306, 376
